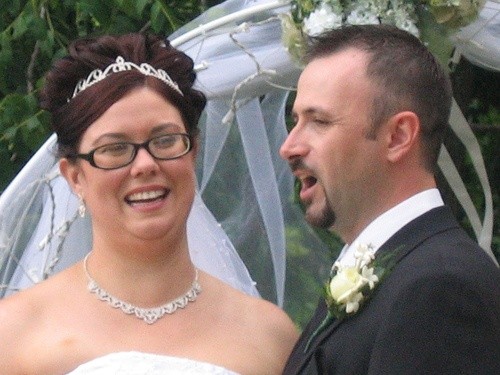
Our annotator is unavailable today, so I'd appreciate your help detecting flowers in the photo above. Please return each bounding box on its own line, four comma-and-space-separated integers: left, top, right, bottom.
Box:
303, 243, 406, 353
277, 0, 487, 72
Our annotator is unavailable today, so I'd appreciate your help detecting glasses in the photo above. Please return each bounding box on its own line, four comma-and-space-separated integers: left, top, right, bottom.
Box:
64, 132, 193, 170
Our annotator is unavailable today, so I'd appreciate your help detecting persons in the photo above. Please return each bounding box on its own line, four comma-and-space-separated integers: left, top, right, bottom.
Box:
0, 33, 302, 375
280, 24, 500, 375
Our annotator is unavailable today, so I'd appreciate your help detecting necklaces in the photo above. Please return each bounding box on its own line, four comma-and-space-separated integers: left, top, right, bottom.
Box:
83, 249, 202, 324
